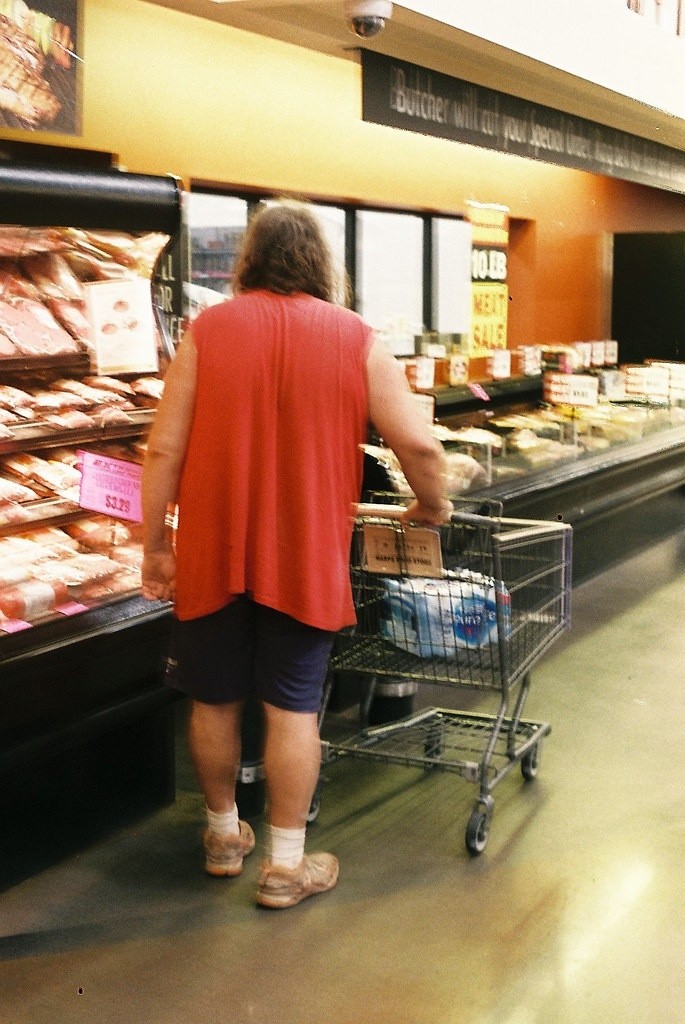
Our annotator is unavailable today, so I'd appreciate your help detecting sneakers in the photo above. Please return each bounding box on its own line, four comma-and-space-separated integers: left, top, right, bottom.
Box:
256, 850, 339, 909
201, 820, 256, 877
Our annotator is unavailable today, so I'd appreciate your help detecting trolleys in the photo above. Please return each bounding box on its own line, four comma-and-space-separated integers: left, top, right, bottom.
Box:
311, 489, 575, 853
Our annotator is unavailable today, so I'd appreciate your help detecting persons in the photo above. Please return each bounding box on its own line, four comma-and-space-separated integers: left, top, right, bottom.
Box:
142, 207, 455, 910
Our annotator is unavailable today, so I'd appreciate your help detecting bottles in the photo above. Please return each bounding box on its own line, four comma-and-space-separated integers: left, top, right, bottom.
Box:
386, 567, 510, 656
